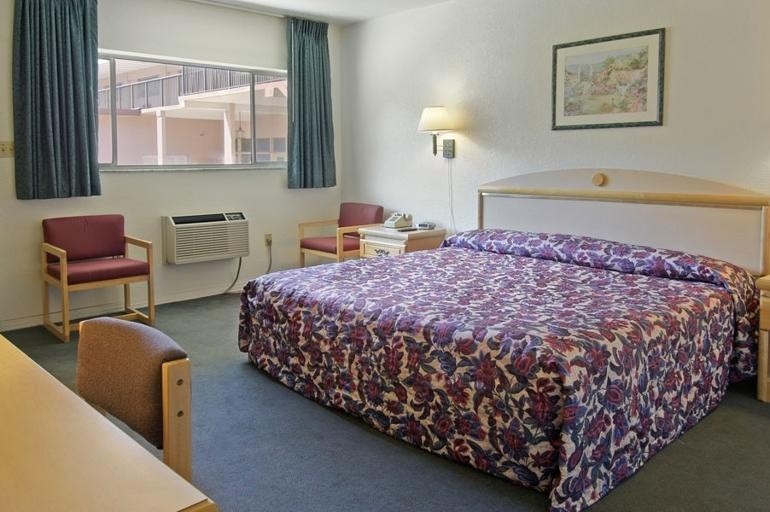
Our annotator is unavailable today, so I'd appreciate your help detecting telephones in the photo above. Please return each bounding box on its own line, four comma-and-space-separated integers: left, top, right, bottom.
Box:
385, 211, 413, 228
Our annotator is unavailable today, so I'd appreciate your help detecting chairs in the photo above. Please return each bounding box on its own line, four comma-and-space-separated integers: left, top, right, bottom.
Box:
76, 316, 192, 484
297, 202, 383, 268
40, 213, 154, 344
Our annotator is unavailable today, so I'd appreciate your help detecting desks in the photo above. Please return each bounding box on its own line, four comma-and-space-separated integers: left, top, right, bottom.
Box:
0, 331, 224, 512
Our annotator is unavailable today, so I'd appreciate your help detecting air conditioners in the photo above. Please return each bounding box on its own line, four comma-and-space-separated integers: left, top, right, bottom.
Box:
161, 210, 249, 265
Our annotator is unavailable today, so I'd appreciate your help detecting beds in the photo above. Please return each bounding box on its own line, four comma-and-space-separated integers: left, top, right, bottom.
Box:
247, 168, 770, 512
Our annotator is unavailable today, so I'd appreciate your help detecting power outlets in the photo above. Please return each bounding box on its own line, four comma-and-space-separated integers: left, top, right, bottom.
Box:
265, 233, 272, 246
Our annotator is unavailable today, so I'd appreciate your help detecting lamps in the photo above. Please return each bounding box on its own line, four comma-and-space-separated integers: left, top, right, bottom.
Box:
418, 107, 466, 157
236, 112, 245, 135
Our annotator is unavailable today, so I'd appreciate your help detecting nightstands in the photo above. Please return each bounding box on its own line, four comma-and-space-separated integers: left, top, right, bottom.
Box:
356, 226, 447, 260
754, 274, 769, 404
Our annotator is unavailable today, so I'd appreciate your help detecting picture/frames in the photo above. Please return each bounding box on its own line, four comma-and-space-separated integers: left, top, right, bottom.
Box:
552, 27, 666, 130
